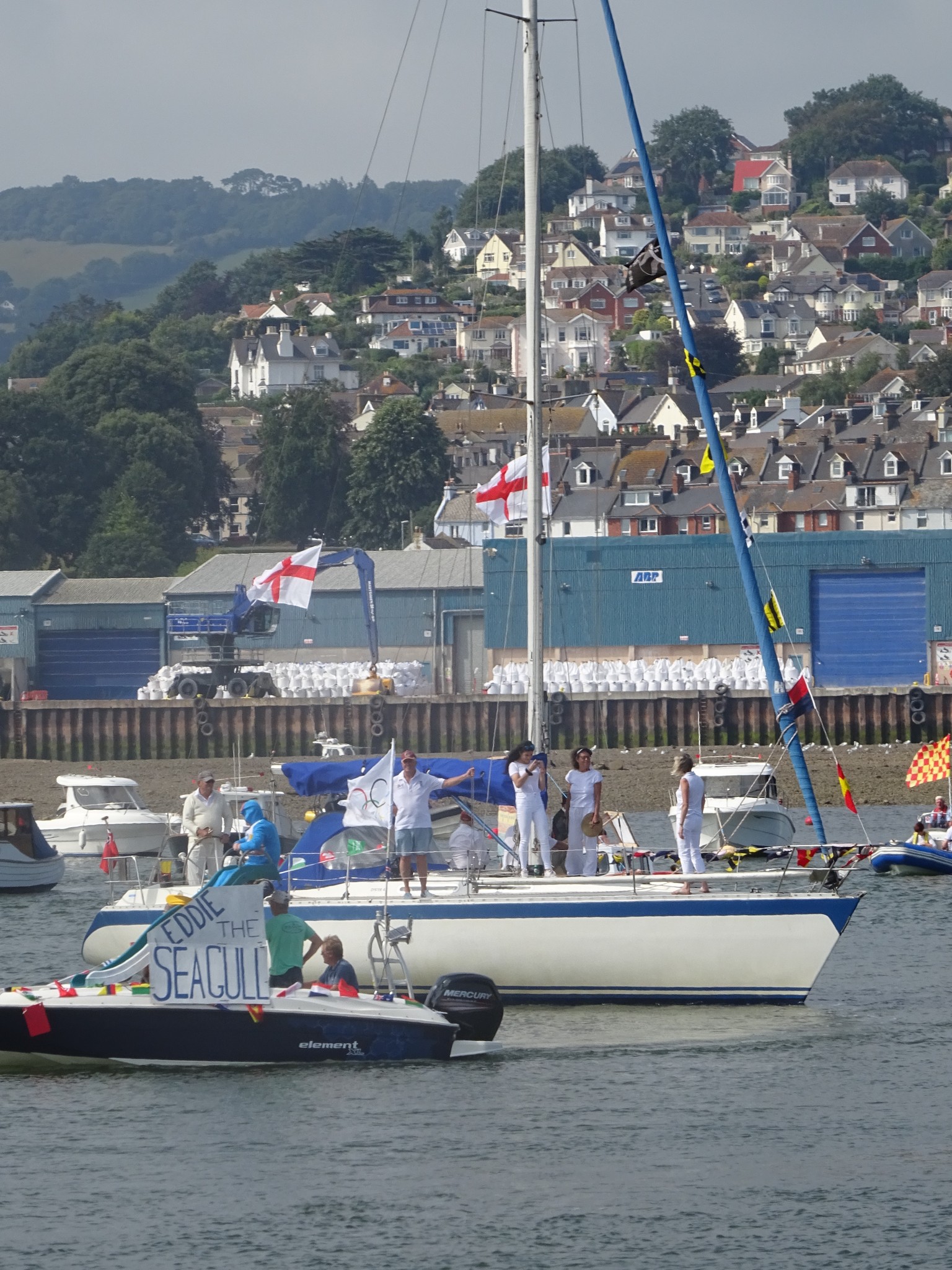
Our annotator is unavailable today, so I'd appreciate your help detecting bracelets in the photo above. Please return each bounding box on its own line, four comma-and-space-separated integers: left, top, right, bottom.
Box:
526, 767, 533, 778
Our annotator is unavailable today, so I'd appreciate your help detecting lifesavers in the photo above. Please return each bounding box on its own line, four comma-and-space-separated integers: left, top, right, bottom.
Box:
197, 711, 208, 724
371, 724, 384, 737
194, 698, 206, 710
715, 683, 727, 695
713, 714, 725, 727
551, 692, 566, 704
909, 687, 924, 699
910, 700, 924, 711
200, 723, 214, 736
370, 695, 384, 710
549, 715, 562, 725
551, 704, 564, 715
715, 701, 726, 714
912, 712, 926, 724
371, 711, 384, 724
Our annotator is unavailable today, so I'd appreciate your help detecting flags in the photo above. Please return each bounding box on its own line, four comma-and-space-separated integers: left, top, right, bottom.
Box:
787, 676, 814, 720
905, 735, 950, 788
474, 444, 552, 523
763, 589, 785, 634
624, 237, 667, 293
100, 828, 121, 875
246, 541, 322, 610
341, 747, 395, 830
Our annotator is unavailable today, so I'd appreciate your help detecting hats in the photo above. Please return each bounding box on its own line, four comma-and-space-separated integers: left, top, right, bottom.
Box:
198, 771, 215, 782
266, 890, 289, 905
581, 812, 603, 837
460, 811, 474, 821
575, 748, 593, 756
560, 791, 568, 804
401, 750, 416, 761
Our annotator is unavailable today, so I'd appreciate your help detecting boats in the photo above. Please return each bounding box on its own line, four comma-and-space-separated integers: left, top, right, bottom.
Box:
868, 731, 952, 878
666, 711, 798, 859
0, 848, 505, 1074
1, 730, 469, 896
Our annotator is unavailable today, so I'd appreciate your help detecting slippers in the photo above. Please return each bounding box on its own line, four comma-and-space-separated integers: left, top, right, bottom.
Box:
671, 889, 691, 895
699, 887, 710, 893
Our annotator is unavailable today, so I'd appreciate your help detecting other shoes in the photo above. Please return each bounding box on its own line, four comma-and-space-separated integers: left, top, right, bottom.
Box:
545, 869, 557, 878
421, 890, 435, 898
521, 868, 529, 877
404, 892, 414, 899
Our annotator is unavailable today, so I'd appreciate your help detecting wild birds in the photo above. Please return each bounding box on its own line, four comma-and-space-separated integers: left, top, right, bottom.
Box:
549, 760, 558, 768
618, 738, 913, 756
244, 752, 255, 760
589, 744, 598, 751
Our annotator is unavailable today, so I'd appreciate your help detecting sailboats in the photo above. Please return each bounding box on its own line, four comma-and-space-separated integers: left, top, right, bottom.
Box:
80, 1, 876, 1007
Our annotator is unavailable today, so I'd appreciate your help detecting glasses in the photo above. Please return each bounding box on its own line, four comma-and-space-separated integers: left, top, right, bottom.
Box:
524, 744, 535, 750
580, 756, 592, 760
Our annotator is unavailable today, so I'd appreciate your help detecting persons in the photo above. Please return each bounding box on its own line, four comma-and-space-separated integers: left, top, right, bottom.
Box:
930, 794, 951, 828
300, 937, 359, 996
203, 800, 281, 888
509, 742, 555, 879
941, 826, 952, 852
551, 790, 571, 848
448, 809, 486, 872
563, 747, 603, 876
393, 749, 473, 898
265, 890, 317, 990
670, 753, 711, 895
912, 823, 935, 849
181, 771, 233, 884
